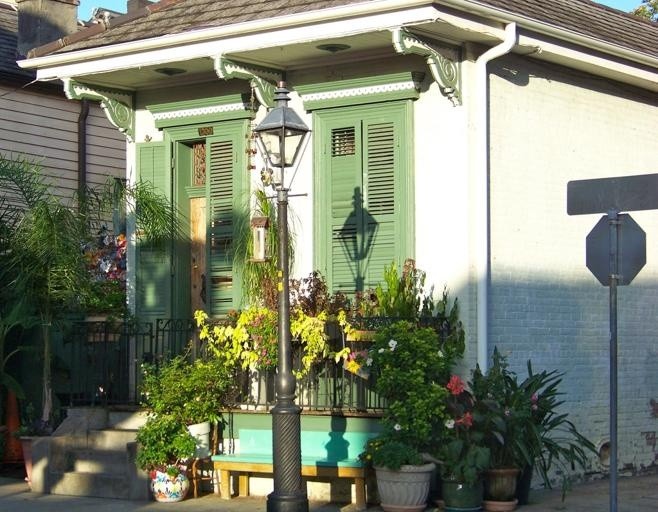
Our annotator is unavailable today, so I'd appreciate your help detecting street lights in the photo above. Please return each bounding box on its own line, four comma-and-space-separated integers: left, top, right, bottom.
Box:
252, 80, 312, 512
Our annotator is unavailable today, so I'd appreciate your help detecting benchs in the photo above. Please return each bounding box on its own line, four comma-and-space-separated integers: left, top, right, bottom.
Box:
210, 454, 368, 509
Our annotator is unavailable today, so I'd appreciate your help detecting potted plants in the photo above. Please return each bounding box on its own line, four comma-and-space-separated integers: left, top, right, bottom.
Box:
134, 340, 234, 503
358, 433, 436, 511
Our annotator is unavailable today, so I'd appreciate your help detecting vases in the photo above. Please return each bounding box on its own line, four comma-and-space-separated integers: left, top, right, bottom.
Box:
483, 467, 520, 511
442, 474, 484, 511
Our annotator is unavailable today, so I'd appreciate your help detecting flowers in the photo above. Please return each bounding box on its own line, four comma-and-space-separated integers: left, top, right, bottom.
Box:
356, 320, 455, 433
468, 347, 601, 503
436, 375, 489, 490
193, 304, 356, 378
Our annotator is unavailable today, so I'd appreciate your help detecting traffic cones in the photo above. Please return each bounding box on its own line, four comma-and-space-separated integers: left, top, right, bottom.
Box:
1, 387, 25, 477
